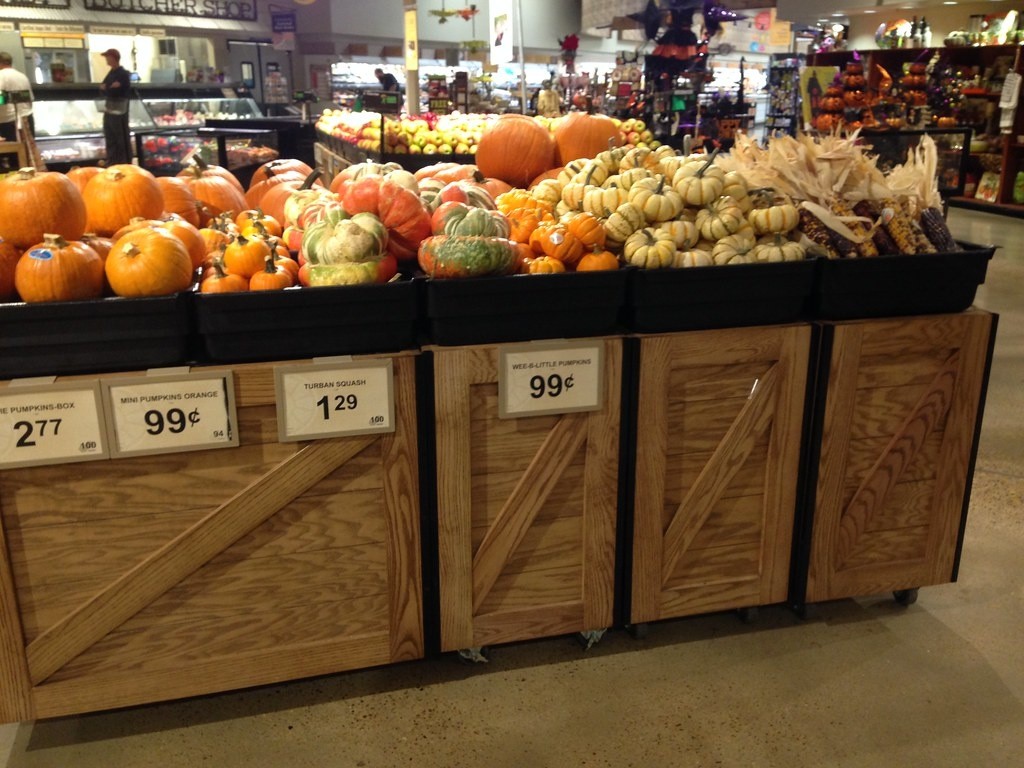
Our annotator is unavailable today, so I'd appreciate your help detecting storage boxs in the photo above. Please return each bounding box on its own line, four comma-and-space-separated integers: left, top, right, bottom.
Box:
0, 127, 997, 383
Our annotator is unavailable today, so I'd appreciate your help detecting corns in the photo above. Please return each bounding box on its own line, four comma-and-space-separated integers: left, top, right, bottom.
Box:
724, 128, 967, 260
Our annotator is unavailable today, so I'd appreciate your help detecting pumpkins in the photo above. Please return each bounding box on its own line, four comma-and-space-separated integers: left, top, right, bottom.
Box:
1, 96, 806, 306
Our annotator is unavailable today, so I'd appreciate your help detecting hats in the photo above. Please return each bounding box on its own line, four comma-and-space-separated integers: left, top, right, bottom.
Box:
101, 49, 120, 61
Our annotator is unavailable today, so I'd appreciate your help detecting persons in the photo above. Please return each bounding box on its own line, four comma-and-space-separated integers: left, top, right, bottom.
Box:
0, 51, 36, 173
374, 69, 401, 92
99, 48, 136, 166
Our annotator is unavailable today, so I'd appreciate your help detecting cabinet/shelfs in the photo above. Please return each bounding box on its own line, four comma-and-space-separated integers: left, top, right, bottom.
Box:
808, 43, 1024, 209
